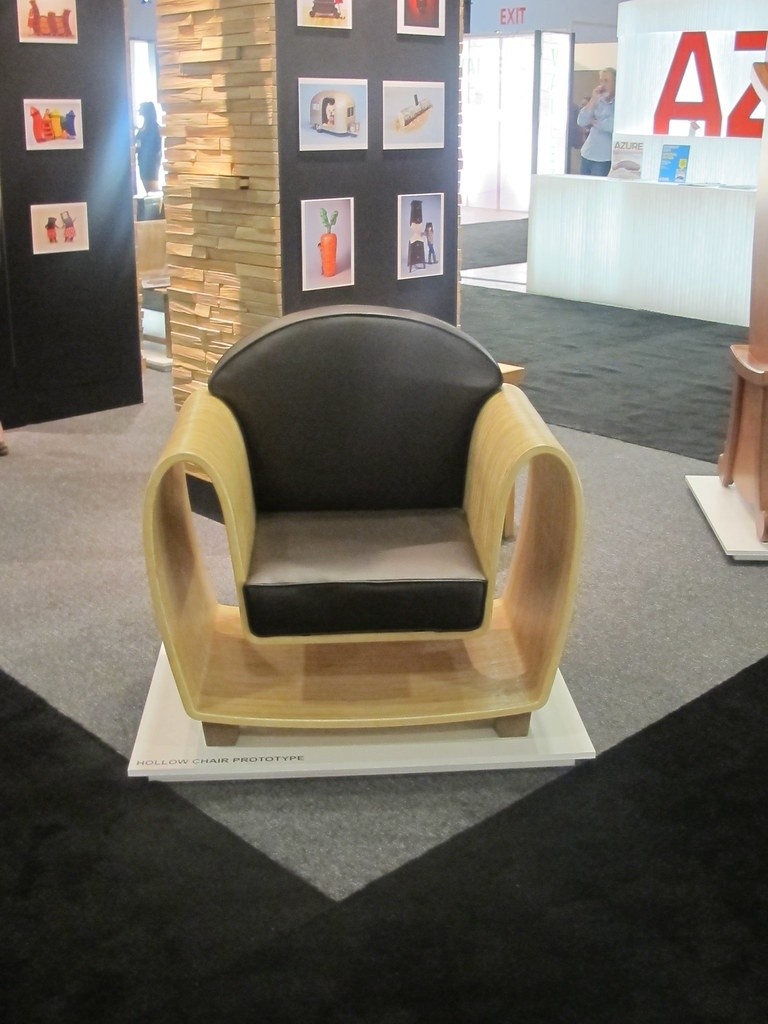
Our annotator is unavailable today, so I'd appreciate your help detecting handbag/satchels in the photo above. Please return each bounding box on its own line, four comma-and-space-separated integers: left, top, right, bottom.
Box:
135, 128, 143, 153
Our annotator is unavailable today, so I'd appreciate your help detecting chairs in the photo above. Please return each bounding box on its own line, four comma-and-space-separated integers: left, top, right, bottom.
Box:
143, 303, 584, 746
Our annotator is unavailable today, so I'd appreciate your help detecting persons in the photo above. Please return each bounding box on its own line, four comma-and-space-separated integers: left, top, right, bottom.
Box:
135, 102, 162, 194
421, 222, 438, 264
577, 68, 616, 176
572, 97, 591, 147
